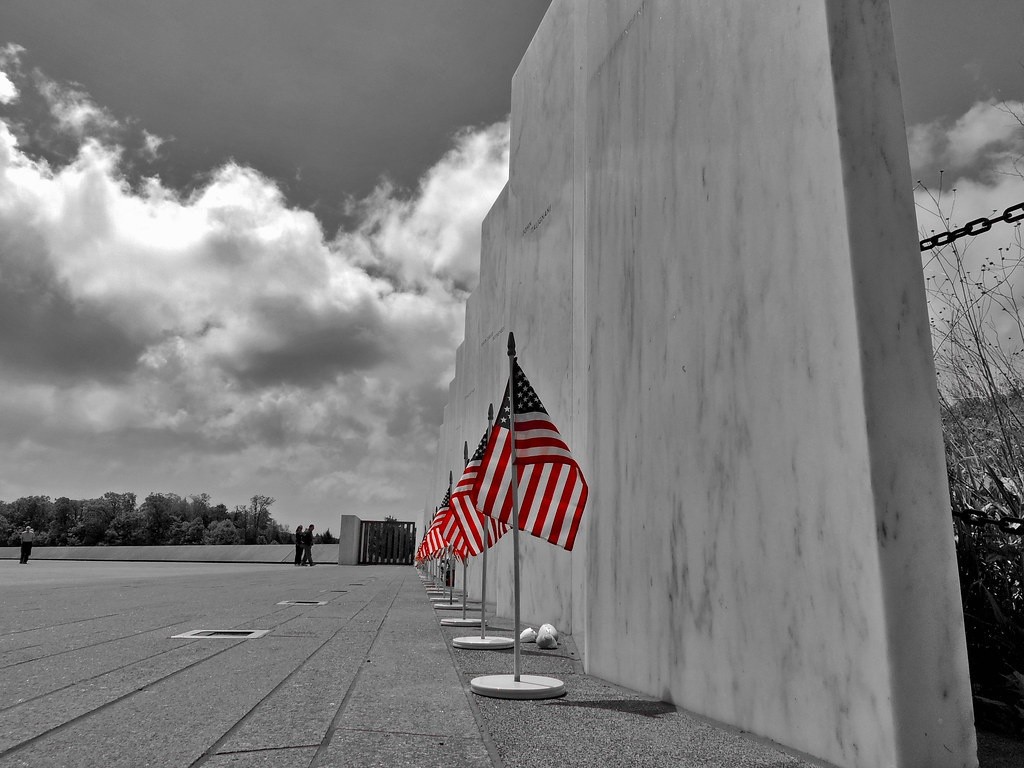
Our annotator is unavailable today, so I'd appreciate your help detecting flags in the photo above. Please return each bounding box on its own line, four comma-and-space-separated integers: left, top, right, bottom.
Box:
470, 358, 588, 550
449, 425, 515, 556
412, 488, 470, 567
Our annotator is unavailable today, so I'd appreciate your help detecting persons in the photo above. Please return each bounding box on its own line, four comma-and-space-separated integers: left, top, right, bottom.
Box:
294, 524, 316, 567
20, 526, 34, 563
